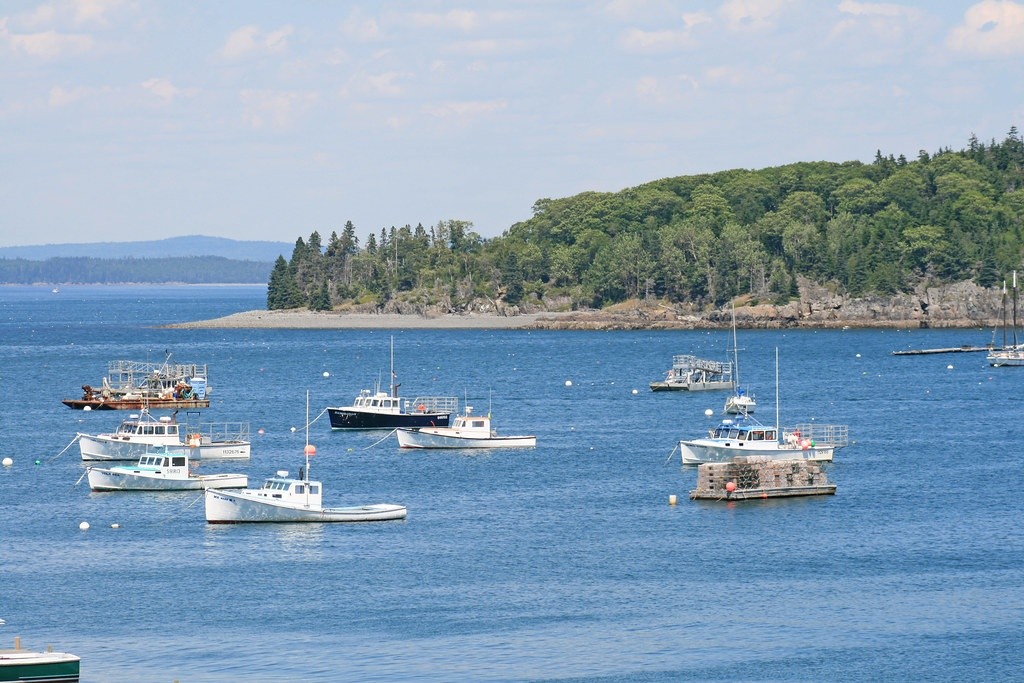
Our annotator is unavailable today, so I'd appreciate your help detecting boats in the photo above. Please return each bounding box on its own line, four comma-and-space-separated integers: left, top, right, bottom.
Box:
206, 389, 406, 523
61, 356, 211, 410
650, 354, 736, 392
326, 335, 458, 430
679, 346, 849, 466
396, 390, 537, 450
86, 395, 248, 492
76, 378, 251, 462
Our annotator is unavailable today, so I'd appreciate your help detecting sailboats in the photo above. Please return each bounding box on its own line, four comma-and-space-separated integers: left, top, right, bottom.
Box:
988, 270, 1024, 367
724, 302, 757, 415
